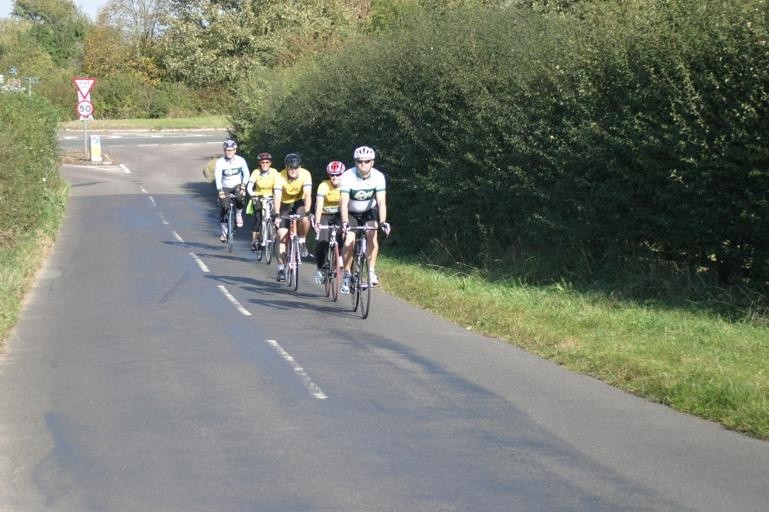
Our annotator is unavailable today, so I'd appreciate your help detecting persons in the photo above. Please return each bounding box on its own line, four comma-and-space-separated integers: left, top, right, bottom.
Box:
272, 153, 311, 282
313, 160, 346, 285
246, 152, 279, 252
339, 145, 391, 294
214, 139, 250, 243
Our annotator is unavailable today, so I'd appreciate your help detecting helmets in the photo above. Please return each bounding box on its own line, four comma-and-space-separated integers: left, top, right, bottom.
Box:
284, 153, 303, 169
256, 152, 272, 160
354, 145, 376, 161
223, 140, 240, 150
326, 161, 348, 176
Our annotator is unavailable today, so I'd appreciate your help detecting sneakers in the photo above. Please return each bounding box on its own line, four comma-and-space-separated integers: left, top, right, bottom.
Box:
277, 259, 286, 275
220, 221, 231, 243
314, 270, 325, 286
342, 271, 352, 296
299, 239, 311, 260
236, 214, 246, 228
369, 270, 380, 288
